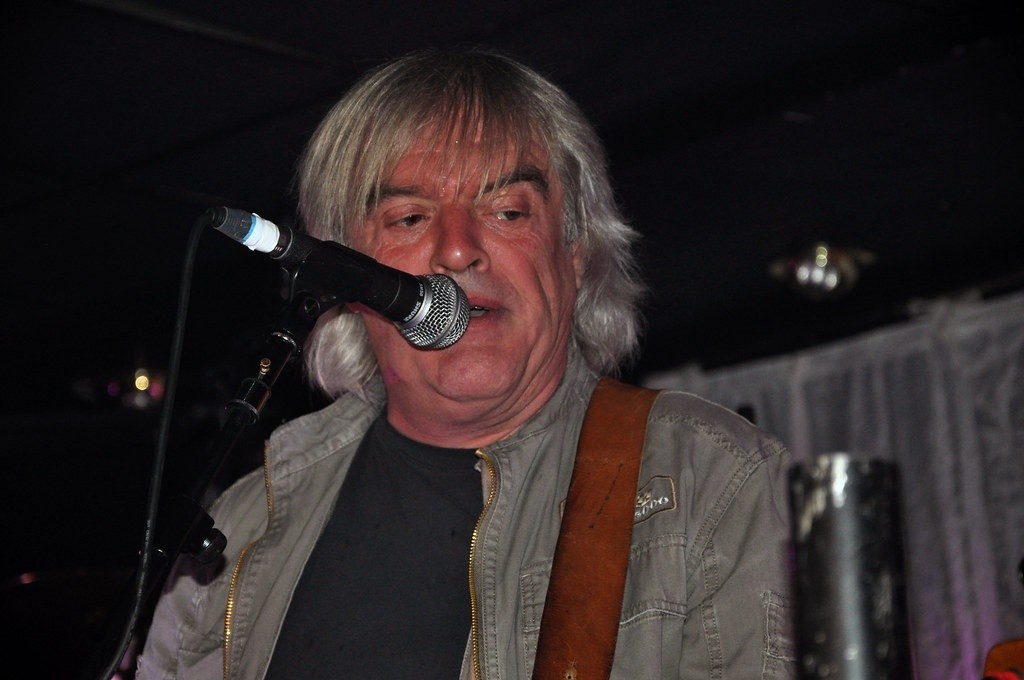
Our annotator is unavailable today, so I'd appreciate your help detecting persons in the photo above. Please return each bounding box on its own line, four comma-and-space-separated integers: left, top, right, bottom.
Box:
136, 47, 793, 680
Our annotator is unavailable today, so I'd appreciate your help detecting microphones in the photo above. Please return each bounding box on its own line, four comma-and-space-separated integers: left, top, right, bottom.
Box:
207, 205, 470, 349
